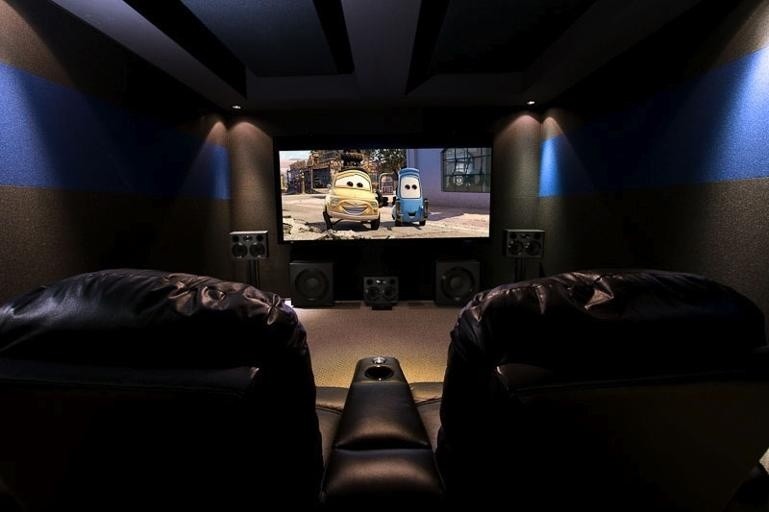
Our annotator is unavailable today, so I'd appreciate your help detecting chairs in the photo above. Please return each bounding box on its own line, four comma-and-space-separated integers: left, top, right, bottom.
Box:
0, 268, 445, 512
321, 271, 769, 512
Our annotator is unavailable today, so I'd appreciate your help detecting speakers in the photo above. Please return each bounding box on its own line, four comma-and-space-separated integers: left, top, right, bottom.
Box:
503, 227, 545, 260
434, 259, 482, 308
363, 275, 399, 310
287, 259, 336, 309
229, 230, 271, 262
